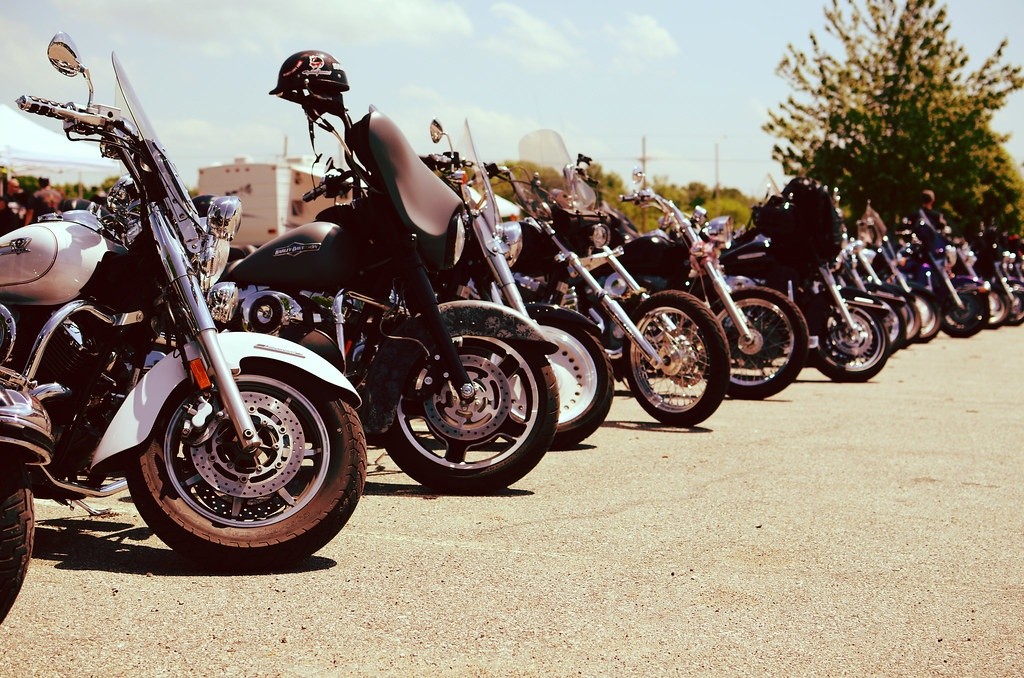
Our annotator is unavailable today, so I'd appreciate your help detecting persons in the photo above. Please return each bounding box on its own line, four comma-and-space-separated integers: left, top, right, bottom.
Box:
0, 178, 37, 236
32, 176, 63, 214
917, 189, 946, 231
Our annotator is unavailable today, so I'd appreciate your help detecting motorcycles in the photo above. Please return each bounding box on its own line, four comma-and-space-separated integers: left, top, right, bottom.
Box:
419, 112, 1023, 429
208, 49, 563, 495
0, 30, 366, 575
0, 306, 52, 625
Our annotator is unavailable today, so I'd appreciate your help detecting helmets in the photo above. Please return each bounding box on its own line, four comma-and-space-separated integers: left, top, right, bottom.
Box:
269, 50, 349, 94
781, 176, 818, 208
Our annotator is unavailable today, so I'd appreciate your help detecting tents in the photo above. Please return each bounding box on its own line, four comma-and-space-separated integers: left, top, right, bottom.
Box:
0, 103, 121, 200
